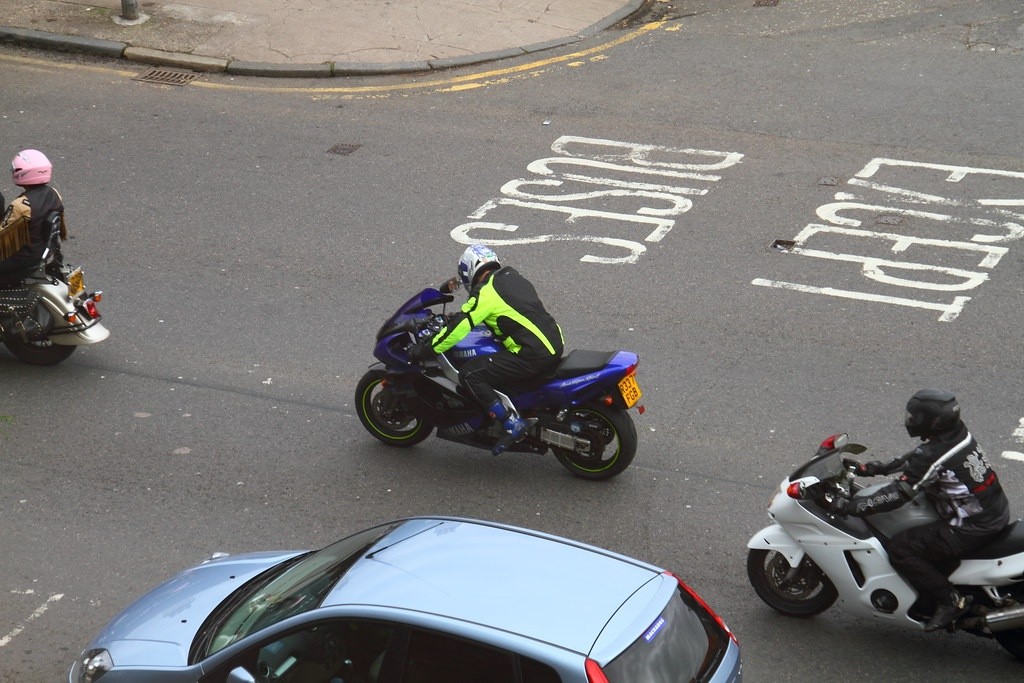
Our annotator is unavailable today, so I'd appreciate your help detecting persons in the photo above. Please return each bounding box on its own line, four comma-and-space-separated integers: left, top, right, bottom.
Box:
0, 149, 65, 273
814, 389, 1009, 632
408, 244, 565, 455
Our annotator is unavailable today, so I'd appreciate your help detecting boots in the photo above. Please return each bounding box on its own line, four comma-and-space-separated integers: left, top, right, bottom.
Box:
483, 395, 528, 456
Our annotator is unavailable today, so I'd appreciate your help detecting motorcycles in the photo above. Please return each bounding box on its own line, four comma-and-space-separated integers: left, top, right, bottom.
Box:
744, 430, 1024, 666
1, 212, 111, 367
352, 278, 646, 481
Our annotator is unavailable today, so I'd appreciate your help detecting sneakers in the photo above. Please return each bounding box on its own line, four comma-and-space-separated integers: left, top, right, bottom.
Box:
923, 587, 965, 633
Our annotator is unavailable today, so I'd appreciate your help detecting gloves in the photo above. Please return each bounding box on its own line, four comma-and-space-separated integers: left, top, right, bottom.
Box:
837, 488, 893, 517
410, 336, 441, 365
856, 458, 903, 478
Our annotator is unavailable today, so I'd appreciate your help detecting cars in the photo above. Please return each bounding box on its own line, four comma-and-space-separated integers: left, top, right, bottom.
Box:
69, 519, 747, 683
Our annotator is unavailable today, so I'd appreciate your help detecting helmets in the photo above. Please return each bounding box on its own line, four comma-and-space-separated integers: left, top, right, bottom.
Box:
904, 387, 960, 439
457, 244, 500, 293
12, 150, 53, 184
